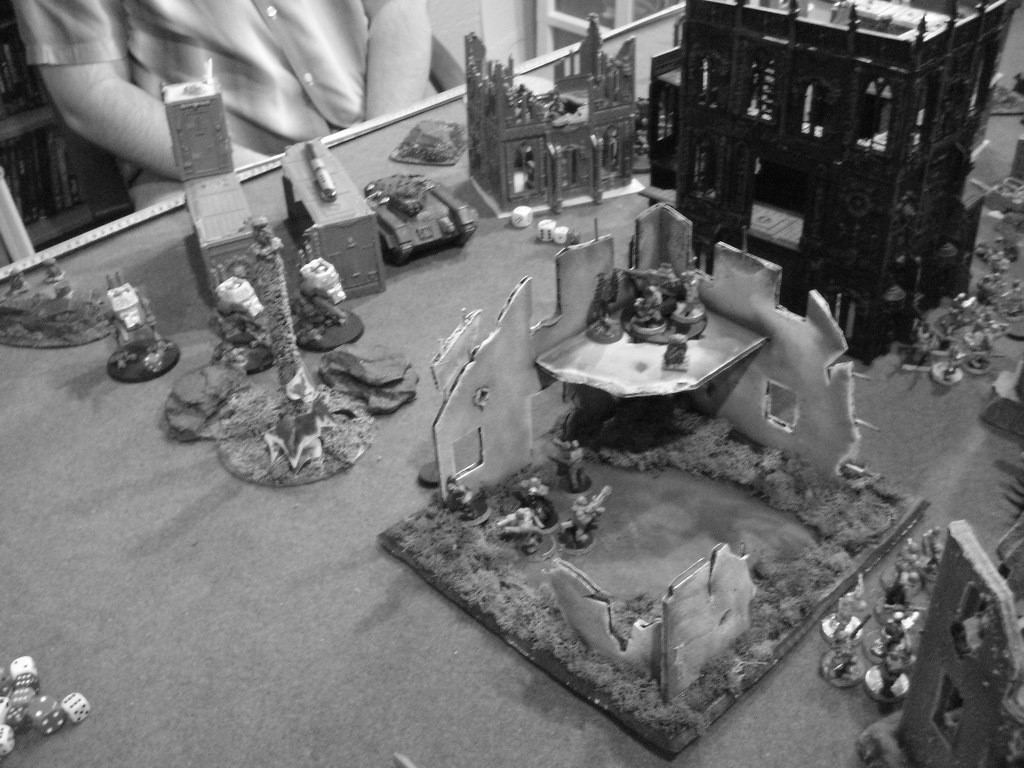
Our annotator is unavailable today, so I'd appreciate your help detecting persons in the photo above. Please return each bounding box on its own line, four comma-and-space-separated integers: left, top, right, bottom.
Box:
10, 0, 435, 215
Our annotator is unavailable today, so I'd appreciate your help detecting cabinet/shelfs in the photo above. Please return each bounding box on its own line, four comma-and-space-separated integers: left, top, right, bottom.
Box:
1, 0, 137, 249
